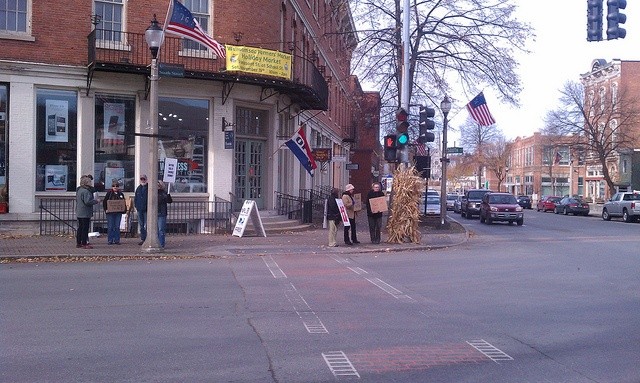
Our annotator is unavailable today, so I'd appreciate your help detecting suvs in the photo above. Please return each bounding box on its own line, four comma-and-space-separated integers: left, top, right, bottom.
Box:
461, 189, 494, 218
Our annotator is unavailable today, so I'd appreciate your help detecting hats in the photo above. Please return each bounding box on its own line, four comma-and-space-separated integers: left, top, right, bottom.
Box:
345, 184, 354, 191
80, 177, 92, 186
158, 180, 165, 189
111, 179, 119, 185
140, 175, 147, 180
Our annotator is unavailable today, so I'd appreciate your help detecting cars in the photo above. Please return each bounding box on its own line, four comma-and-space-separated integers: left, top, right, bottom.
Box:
515, 195, 531, 209
419, 195, 448, 218
420, 190, 440, 195
453, 195, 464, 213
537, 195, 562, 212
480, 191, 524, 225
553, 196, 590, 216
445, 193, 458, 211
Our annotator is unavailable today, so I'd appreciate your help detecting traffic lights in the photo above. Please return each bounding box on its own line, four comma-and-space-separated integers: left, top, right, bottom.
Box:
395, 108, 410, 146
419, 106, 435, 143
383, 134, 396, 161
606, 0, 627, 40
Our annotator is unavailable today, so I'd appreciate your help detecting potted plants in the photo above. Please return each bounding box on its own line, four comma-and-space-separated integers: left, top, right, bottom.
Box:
0, 185, 7, 213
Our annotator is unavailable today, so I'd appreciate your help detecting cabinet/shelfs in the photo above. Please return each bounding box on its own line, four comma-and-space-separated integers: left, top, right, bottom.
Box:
158, 136, 205, 192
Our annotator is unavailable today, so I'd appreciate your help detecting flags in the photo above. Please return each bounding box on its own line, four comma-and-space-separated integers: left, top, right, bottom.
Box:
466, 91, 495, 126
283, 126, 317, 177
554, 152, 563, 164
164, 0, 227, 59
412, 138, 427, 157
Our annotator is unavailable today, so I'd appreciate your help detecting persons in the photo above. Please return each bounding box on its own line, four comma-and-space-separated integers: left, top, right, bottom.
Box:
342, 183, 360, 245
134, 175, 148, 245
366, 183, 384, 244
103, 180, 126, 244
326, 188, 342, 247
158, 179, 172, 246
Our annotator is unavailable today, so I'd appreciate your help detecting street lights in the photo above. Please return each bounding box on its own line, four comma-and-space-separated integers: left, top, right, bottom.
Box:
138, 11, 167, 254
437, 92, 452, 230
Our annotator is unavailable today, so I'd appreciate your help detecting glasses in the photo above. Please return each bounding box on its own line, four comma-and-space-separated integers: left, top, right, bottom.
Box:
112, 185, 119, 188
140, 178, 145, 180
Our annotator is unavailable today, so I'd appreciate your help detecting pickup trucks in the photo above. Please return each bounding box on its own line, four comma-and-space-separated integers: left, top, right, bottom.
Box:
602, 191, 640, 222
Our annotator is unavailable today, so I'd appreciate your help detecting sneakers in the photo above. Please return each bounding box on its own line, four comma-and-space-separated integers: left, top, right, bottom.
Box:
82, 245, 93, 249
138, 241, 145, 245
76, 245, 82, 248
345, 241, 353, 245
354, 240, 360, 243
376, 240, 379, 243
115, 242, 119, 244
372, 241, 375, 243
108, 241, 112, 244
